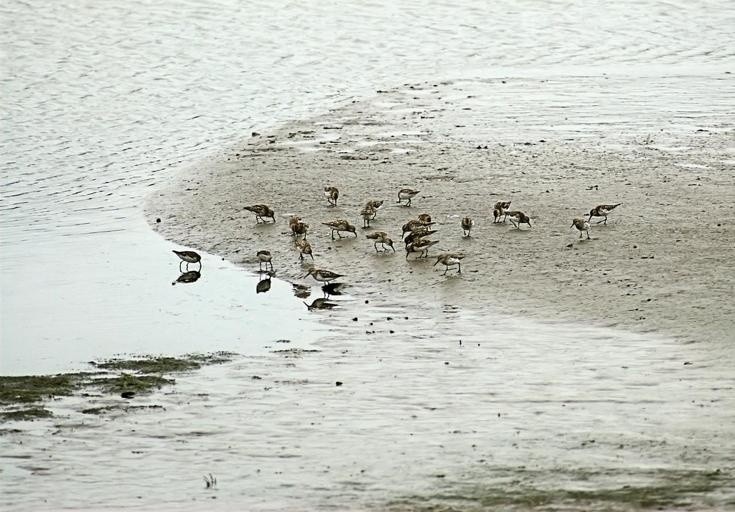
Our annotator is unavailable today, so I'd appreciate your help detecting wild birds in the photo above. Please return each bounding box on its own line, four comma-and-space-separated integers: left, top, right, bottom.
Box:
492, 200, 532, 231
173, 250, 201, 272
434, 253, 465, 276
302, 288, 337, 312
304, 268, 348, 286
461, 218, 474, 238
588, 204, 622, 225
289, 187, 439, 261
171, 266, 202, 286
571, 219, 591, 239
255, 271, 273, 294
243, 205, 275, 224
257, 251, 272, 270
